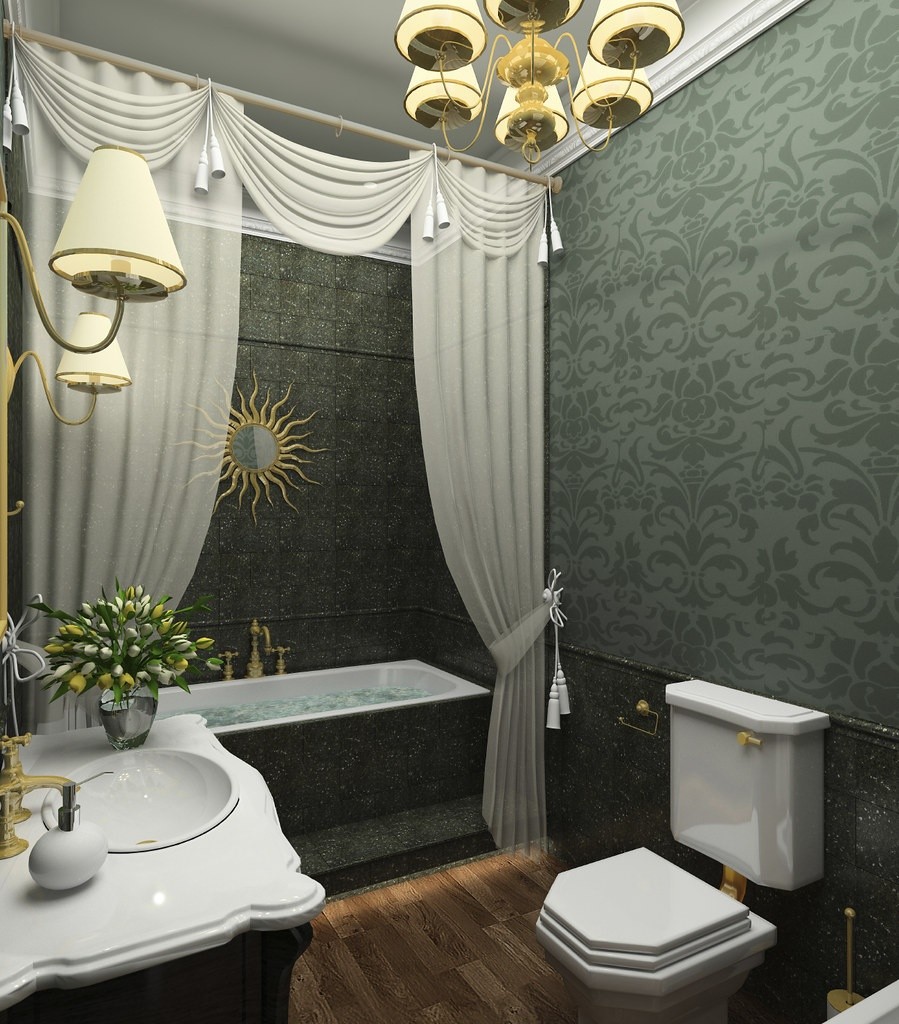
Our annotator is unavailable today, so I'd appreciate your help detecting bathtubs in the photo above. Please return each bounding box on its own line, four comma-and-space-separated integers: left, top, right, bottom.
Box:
153, 658, 497, 832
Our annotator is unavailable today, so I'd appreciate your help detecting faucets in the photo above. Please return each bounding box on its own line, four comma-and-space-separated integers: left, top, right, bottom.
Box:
0, 733, 81, 825
246, 619, 273, 678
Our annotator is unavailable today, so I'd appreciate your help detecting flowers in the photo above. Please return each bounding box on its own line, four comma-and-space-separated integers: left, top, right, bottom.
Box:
25, 577, 224, 713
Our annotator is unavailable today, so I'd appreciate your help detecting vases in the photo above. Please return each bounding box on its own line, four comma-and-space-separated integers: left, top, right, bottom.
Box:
97, 680, 158, 754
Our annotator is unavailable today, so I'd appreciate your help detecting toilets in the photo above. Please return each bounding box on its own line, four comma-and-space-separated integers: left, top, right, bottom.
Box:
531, 678, 827, 1024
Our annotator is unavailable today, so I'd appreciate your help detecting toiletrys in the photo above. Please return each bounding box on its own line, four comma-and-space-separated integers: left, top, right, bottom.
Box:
30, 771, 115, 889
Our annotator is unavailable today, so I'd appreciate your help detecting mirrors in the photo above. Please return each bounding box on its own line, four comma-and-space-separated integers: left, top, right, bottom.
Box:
170, 368, 334, 529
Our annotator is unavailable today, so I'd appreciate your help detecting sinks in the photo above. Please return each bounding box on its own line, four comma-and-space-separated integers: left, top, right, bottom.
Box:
43, 747, 238, 854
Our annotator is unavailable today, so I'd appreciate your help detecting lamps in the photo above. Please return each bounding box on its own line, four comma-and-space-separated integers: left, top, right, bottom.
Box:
393, 1, 685, 165
6, 311, 133, 427
0, 144, 187, 356
